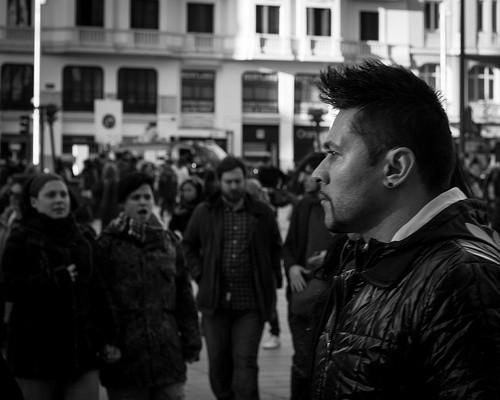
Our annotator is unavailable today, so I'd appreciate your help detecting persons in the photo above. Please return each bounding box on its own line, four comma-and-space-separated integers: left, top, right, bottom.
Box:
245, 179, 284, 349
0, 143, 499, 274
281, 151, 345, 400
93, 170, 204, 399
313, 56, 499, 400
180, 154, 284, 400
0, 174, 101, 400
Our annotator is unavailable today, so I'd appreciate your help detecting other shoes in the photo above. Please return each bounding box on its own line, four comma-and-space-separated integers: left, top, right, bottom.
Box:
263, 335, 281, 349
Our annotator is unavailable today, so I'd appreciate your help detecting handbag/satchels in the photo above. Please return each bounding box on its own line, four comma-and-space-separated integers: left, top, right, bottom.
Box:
291, 266, 331, 316
